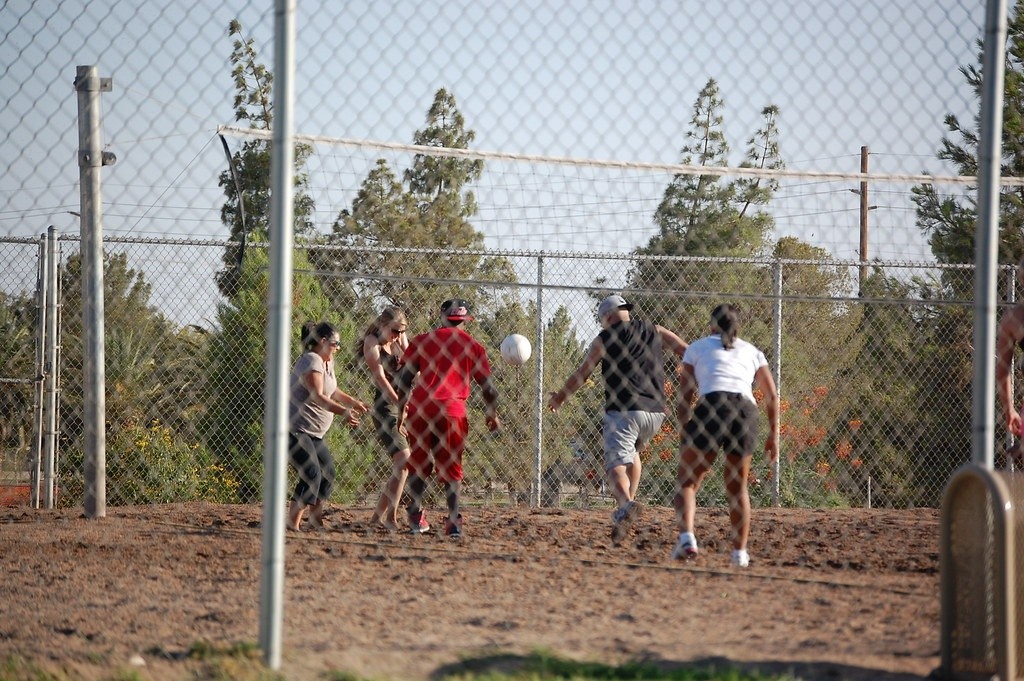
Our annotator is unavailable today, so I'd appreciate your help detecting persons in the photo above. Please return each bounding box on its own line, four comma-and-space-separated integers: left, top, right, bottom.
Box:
396, 299, 501, 539
286, 319, 371, 533
994, 258, 1024, 472
547, 294, 698, 545
355, 305, 420, 533
671, 302, 779, 569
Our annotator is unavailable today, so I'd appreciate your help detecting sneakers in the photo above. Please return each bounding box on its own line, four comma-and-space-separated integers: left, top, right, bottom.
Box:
406, 506, 429, 533
672, 532, 698, 561
443, 514, 462, 540
730, 550, 750, 569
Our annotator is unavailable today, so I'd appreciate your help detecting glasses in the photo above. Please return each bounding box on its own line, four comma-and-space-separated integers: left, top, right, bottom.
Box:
391, 328, 406, 335
326, 337, 340, 347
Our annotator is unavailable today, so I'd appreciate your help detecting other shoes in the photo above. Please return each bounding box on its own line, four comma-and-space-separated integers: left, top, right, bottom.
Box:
611, 499, 644, 544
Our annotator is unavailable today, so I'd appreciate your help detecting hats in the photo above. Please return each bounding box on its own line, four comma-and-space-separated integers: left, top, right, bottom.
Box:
598, 295, 634, 320
441, 298, 473, 321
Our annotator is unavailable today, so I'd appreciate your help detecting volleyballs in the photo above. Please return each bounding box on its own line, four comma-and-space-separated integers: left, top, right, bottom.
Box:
501, 333, 531, 365
